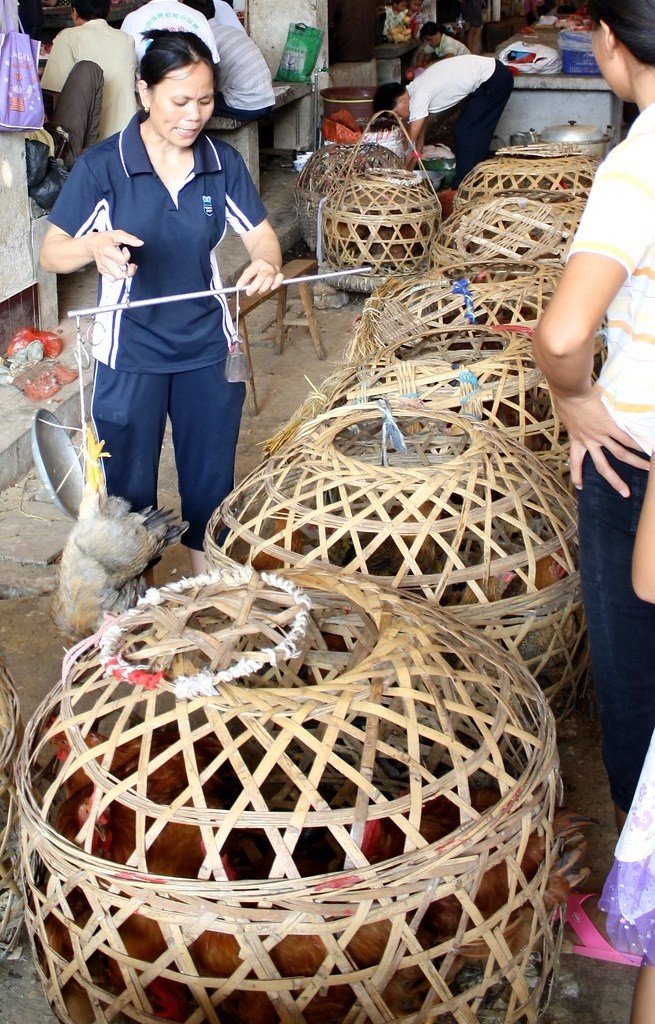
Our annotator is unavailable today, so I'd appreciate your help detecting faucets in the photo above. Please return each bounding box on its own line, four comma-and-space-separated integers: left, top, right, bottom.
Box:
320, 60, 330, 77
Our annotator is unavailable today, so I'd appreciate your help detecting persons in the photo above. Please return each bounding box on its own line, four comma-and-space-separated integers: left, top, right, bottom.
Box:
532, 0, 654, 966
39, 28, 284, 597
376, 0, 486, 70
17, 0, 276, 173
593, 435, 654, 1024
372, 54, 515, 191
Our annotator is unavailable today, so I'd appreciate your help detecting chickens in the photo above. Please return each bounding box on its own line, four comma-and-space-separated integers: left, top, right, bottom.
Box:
42, 713, 599, 1024
47, 428, 188, 660
241, 490, 580, 607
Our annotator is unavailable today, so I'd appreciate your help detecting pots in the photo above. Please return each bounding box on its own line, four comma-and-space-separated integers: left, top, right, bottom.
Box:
529, 120, 615, 160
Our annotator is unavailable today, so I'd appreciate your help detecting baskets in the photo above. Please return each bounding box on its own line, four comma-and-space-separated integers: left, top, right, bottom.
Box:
560, 30, 602, 76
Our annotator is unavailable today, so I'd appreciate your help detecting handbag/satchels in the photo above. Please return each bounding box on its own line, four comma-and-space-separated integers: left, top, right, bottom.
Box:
0, 0, 45, 132
499, 40, 563, 76
275, 21, 323, 86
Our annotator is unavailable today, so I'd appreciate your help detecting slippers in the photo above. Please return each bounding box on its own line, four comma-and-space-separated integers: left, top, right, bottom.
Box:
549, 893, 647, 967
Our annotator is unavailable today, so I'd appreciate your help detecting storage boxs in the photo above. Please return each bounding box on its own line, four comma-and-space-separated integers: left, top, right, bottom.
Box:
559, 20, 602, 75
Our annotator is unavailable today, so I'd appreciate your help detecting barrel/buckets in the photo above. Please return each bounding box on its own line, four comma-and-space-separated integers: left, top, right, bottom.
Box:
320, 85, 377, 119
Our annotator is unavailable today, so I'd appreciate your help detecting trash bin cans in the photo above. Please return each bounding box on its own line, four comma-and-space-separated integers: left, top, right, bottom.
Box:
319, 86, 378, 132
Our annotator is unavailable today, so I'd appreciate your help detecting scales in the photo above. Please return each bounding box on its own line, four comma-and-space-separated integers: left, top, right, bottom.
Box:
30, 261, 377, 522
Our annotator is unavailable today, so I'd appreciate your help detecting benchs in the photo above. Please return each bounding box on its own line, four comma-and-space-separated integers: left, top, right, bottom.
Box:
207, 80, 316, 198
375, 38, 421, 84
229, 257, 331, 415
